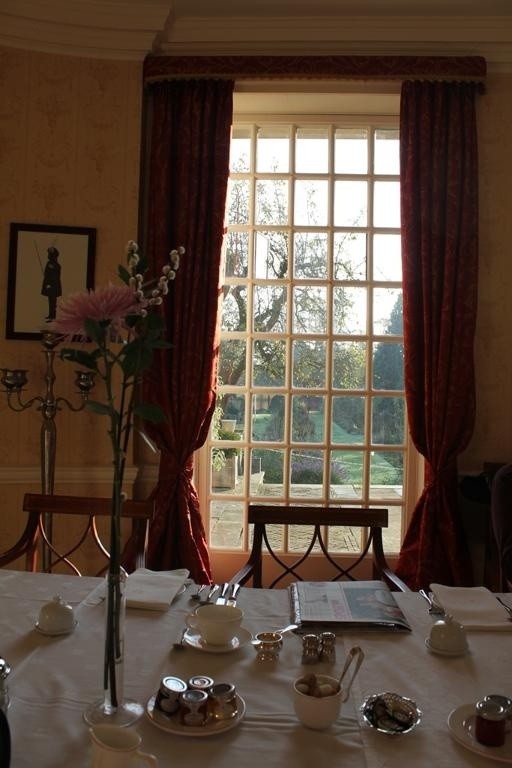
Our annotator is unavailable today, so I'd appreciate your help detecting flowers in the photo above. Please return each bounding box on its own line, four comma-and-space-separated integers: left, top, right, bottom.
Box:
54, 240, 188, 707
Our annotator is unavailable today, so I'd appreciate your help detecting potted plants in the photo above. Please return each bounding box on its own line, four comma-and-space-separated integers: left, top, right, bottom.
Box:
212, 430, 241, 490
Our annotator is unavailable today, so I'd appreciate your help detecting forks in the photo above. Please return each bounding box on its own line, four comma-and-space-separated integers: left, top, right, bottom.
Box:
416, 589, 445, 615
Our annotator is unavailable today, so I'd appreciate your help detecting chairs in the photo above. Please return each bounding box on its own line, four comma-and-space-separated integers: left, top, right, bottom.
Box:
491, 462, 512, 592
227, 504, 413, 593
0, 493, 155, 574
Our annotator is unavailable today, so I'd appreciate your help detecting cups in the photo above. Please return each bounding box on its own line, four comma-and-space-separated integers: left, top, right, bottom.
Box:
294, 674, 339, 731
88, 721, 158, 768
186, 604, 244, 645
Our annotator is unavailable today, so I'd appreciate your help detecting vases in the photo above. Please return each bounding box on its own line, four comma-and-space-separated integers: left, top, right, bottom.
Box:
220, 418, 237, 434
82, 565, 145, 727
243, 455, 261, 473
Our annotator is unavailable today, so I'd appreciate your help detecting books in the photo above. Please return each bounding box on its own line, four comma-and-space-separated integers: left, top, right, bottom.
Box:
289, 579, 411, 636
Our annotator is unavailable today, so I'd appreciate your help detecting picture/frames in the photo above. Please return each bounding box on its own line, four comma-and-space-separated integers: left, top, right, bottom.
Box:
6, 222, 96, 342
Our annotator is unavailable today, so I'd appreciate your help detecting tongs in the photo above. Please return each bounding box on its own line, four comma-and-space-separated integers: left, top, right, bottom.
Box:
337, 644, 364, 703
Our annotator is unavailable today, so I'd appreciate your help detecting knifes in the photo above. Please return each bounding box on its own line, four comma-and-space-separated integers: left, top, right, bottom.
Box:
214, 581, 240, 606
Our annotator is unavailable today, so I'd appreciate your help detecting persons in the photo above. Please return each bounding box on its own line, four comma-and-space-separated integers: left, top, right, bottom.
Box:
40, 245, 64, 324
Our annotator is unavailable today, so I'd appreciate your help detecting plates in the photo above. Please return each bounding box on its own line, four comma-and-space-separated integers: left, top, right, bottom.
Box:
425, 636, 472, 656
32, 618, 81, 635
445, 702, 512, 766
184, 627, 252, 655
146, 689, 246, 737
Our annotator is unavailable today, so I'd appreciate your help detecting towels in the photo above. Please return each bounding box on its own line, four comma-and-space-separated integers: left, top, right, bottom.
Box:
429, 581, 512, 630
121, 567, 190, 611
